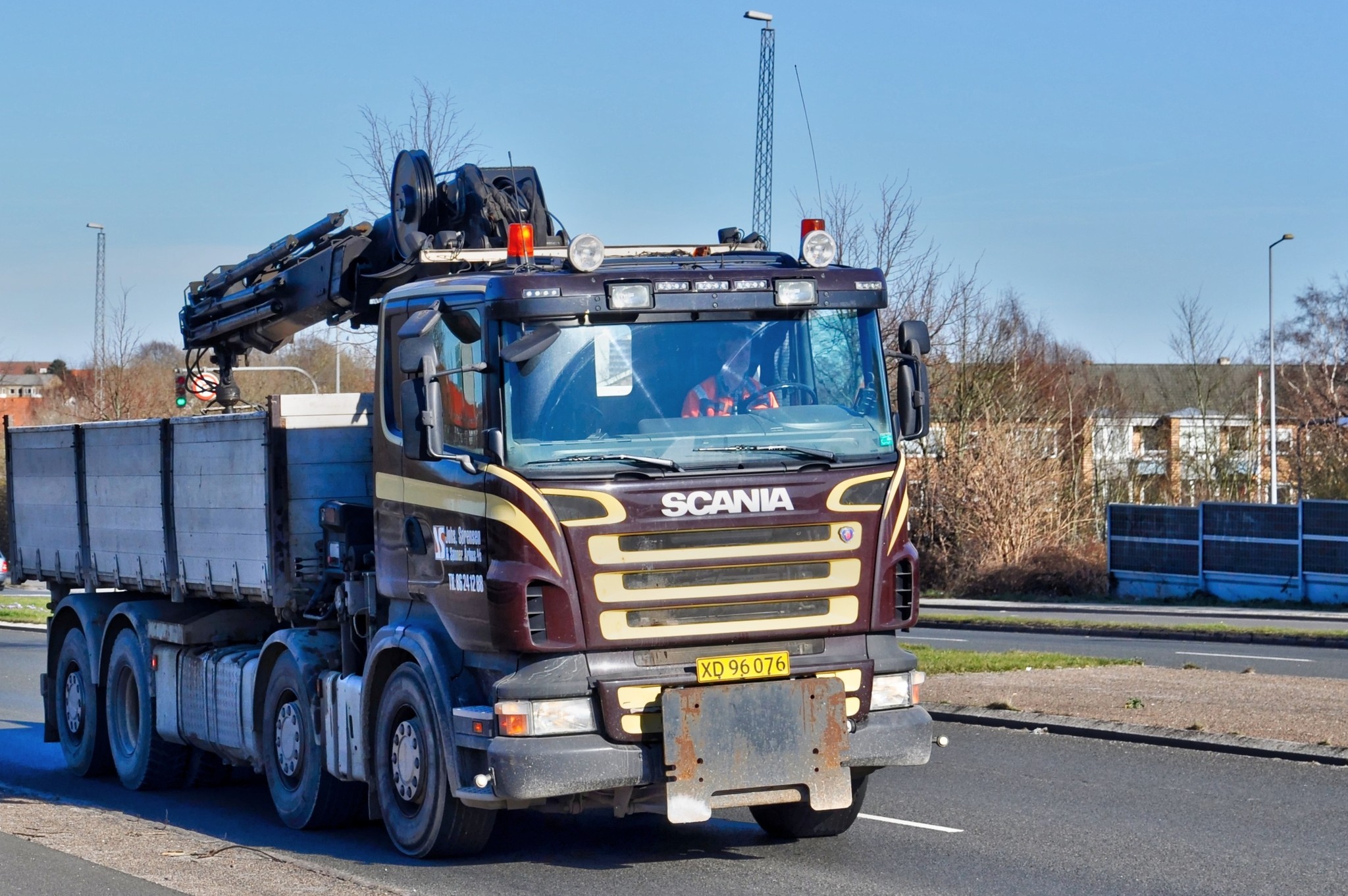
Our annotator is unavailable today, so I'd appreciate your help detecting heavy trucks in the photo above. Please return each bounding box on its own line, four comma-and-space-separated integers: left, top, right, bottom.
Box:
0, 147, 950, 864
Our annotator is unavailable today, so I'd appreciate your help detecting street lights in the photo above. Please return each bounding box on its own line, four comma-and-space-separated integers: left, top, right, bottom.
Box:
1268, 233, 1296, 505
744, 11, 776, 251
84, 223, 107, 420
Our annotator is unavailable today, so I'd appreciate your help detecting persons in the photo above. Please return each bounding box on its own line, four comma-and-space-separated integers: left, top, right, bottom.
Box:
680, 329, 780, 419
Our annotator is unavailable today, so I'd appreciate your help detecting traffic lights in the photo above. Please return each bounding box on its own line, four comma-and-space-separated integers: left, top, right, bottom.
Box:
175, 373, 187, 409
